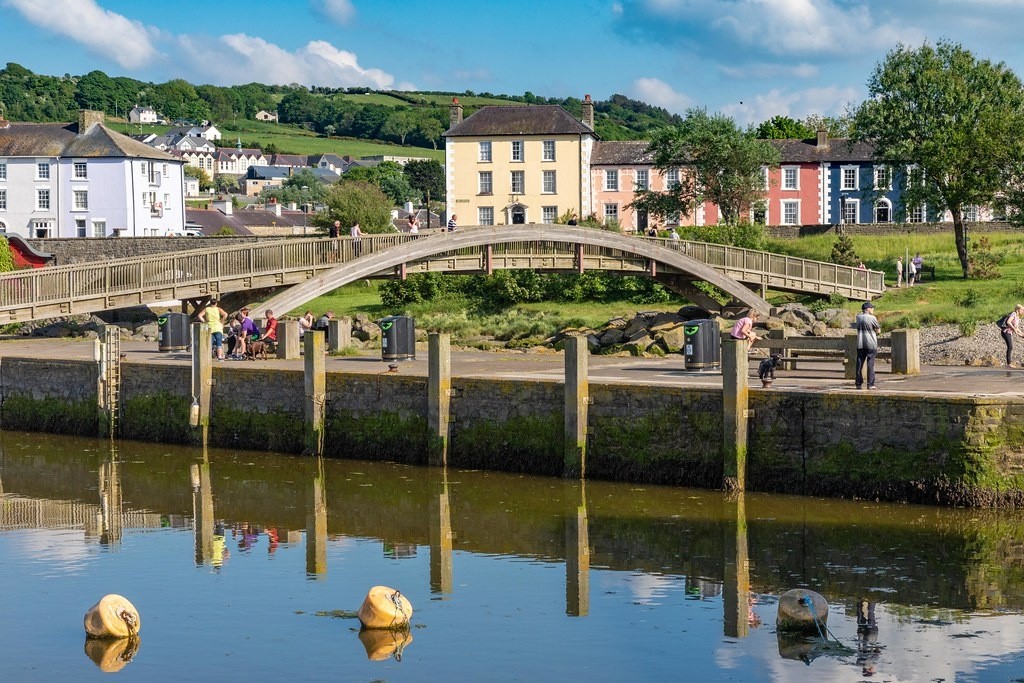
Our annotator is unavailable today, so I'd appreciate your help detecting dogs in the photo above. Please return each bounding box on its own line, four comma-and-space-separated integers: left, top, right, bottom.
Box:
248, 341, 269, 361
757, 355, 782, 379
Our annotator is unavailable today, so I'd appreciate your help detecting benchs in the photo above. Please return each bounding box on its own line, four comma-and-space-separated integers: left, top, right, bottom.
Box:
219, 317, 355, 359
721, 331, 868, 381
770, 327, 920, 375
903, 266, 936, 280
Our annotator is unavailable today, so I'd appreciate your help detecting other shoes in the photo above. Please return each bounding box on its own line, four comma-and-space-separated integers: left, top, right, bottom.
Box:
225, 353, 229, 357
218, 358, 224, 361
235, 355, 247, 360
867, 385, 876, 390
856, 385, 861, 389
1007, 364, 1016, 368
228, 354, 238, 359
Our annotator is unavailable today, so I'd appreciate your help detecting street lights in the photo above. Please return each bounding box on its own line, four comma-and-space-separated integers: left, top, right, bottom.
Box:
300, 185, 311, 240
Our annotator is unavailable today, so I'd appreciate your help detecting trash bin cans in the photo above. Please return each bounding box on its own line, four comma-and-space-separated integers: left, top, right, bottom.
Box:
381, 317, 415, 361
158, 313, 190, 351
684, 319, 720, 371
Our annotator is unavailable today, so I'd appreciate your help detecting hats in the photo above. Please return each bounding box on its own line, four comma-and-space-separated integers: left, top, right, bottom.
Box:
862, 302, 876, 310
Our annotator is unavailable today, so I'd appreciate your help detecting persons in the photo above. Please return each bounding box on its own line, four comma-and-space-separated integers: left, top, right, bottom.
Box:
267, 197, 273, 203
197, 299, 228, 361
257, 202, 264, 208
407, 215, 419, 239
730, 308, 763, 354
856, 262, 866, 281
914, 252, 922, 282
568, 214, 578, 226
897, 256, 903, 288
648, 225, 690, 253
909, 258, 917, 287
350, 222, 368, 258
323, 221, 341, 263
225, 309, 260, 360
448, 214, 457, 232
258, 310, 277, 343
856, 302, 881, 390
316, 311, 333, 338
1001, 304, 1024, 368
299, 310, 313, 338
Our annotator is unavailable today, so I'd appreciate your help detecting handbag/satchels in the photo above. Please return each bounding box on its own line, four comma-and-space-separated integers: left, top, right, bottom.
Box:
996, 311, 1017, 330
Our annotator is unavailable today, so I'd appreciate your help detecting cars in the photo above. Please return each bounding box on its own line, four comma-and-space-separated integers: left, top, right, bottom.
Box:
187, 230, 206, 237
